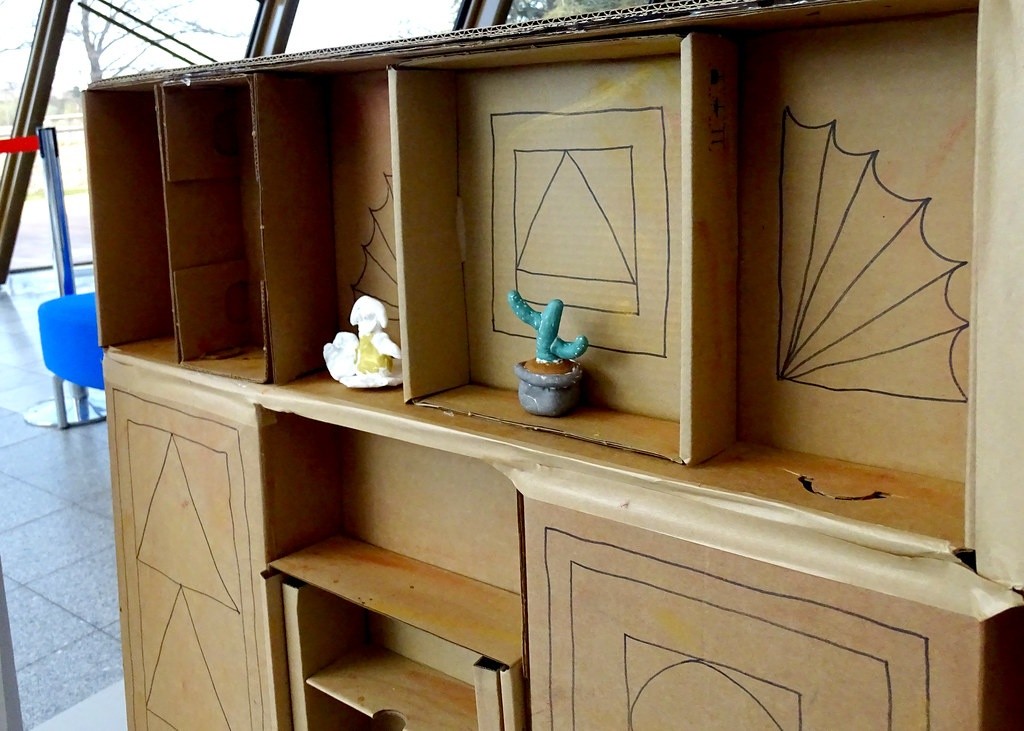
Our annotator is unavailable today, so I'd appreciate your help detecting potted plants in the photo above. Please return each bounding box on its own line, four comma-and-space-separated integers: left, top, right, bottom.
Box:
507, 290, 588, 416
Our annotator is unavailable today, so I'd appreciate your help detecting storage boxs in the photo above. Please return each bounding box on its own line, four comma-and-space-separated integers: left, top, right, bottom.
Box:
83, 0, 1024, 731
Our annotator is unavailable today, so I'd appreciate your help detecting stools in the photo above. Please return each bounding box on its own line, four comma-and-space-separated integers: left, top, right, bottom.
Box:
38, 291, 107, 429
